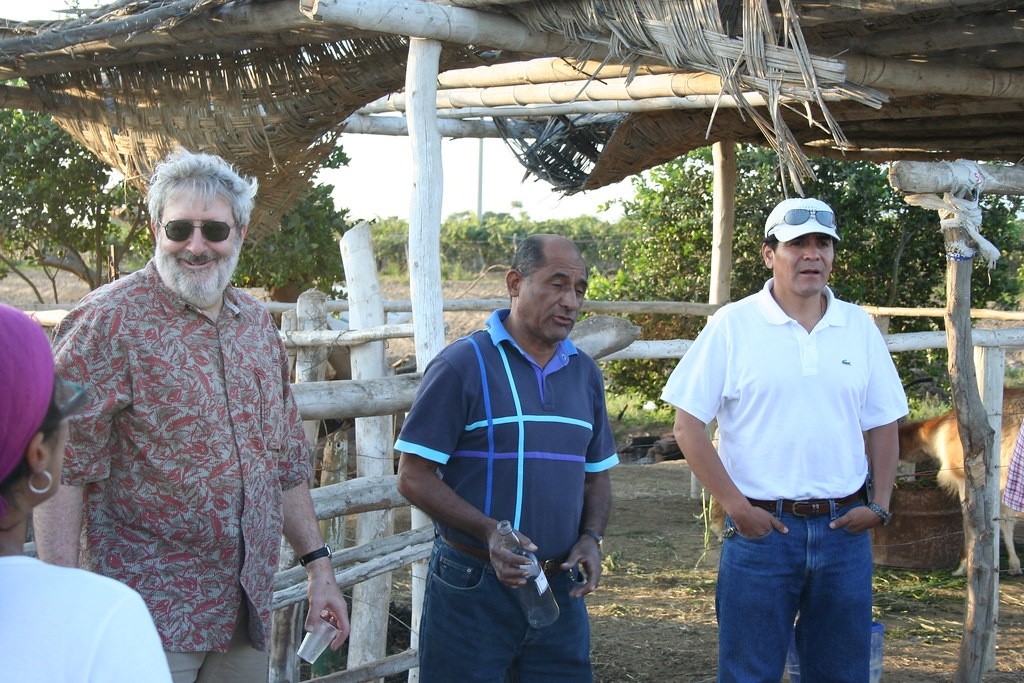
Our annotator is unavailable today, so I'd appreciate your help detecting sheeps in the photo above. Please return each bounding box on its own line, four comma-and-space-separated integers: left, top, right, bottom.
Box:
862, 375, 1024, 578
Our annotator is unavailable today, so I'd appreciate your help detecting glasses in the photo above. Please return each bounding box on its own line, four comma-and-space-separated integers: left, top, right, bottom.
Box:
157, 220, 238, 242
766, 209, 837, 238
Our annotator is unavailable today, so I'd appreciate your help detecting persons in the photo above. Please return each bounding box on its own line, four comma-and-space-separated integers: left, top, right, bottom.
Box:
660, 197, 910, 683
394, 234, 620, 683
0, 303, 177, 683
33, 145, 350, 683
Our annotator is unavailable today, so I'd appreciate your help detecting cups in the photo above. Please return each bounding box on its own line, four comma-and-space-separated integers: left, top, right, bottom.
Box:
297, 619, 337, 664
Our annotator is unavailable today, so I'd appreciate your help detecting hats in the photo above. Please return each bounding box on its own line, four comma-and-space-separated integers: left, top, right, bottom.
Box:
765, 198, 841, 243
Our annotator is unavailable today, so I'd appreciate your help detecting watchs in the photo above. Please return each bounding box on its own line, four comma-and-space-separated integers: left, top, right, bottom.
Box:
868, 501, 892, 527
299, 543, 333, 566
582, 529, 605, 556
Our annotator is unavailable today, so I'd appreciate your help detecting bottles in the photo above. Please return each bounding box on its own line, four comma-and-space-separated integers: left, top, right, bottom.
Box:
498, 520, 559, 627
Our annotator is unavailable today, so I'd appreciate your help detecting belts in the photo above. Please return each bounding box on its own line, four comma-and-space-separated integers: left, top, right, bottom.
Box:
750, 484, 866, 518
441, 535, 565, 577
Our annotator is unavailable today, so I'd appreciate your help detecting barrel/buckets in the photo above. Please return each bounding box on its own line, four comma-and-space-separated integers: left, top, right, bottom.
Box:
870, 483, 965, 569
786, 623, 884, 683
